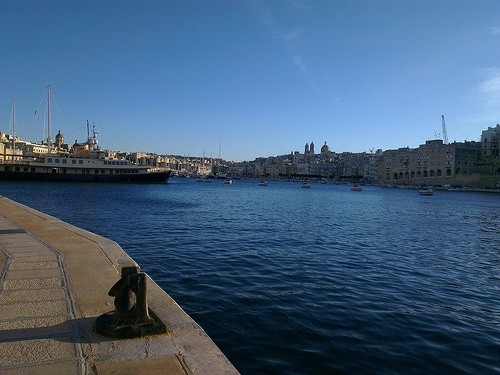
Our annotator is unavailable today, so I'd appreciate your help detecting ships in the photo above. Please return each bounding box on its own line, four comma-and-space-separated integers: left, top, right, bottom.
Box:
0, 144, 172, 185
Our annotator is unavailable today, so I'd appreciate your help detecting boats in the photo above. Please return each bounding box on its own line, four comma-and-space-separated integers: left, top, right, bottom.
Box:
351, 186, 362, 191
300, 182, 311, 187
258, 181, 267, 185
419, 190, 433, 195
223, 179, 232, 184
